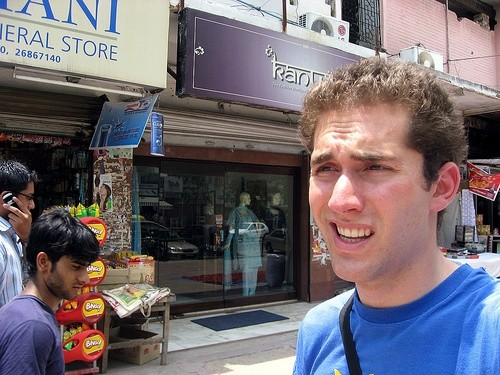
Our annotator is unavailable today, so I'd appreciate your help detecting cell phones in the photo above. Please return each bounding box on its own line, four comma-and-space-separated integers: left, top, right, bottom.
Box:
2, 193, 23, 216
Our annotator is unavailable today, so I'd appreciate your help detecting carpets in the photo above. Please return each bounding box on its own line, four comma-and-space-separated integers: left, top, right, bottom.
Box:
177, 288, 252, 300
183, 269, 267, 286
191, 309, 289, 332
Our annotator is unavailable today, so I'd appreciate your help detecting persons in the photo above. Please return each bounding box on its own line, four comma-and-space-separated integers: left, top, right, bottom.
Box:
223, 192, 288, 293
0, 157, 35, 307
291, 57, 500, 375
99, 185, 112, 208
0, 208, 98, 375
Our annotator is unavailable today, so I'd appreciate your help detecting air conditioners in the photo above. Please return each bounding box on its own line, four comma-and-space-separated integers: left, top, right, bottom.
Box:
297, 12, 350, 43
398, 47, 443, 72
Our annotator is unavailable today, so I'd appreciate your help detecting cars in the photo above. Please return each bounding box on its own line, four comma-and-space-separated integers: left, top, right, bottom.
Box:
230, 222, 269, 242
263, 228, 286, 254
178, 224, 225, 254
131, 215, 198, 261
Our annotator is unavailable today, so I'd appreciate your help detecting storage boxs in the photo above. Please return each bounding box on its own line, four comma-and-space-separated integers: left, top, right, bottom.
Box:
108, 328, 161, 365
98, 261, 155, 293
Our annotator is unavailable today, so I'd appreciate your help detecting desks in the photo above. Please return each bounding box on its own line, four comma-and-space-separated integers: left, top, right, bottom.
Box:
100, 292, 176, 374
445, 252, 500, 277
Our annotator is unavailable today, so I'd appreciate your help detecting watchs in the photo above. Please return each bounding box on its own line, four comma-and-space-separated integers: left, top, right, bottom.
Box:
18, 239, 30, 246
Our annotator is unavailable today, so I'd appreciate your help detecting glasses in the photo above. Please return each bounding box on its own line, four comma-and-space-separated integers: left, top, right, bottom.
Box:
7, 190, 36, 202
